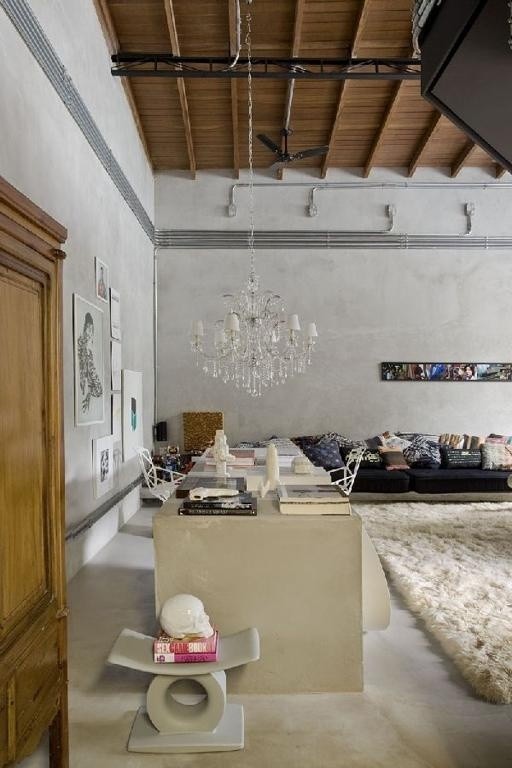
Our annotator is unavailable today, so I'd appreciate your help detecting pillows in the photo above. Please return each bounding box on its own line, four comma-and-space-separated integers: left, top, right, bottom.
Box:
303, 433, 512, 471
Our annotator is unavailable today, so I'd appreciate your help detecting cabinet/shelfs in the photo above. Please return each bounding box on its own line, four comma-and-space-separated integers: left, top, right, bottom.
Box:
0, 176, 70, 767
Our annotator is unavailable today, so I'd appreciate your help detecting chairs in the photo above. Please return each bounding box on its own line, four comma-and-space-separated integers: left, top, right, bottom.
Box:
134, 446, 186, 502
328, 448, 365, 497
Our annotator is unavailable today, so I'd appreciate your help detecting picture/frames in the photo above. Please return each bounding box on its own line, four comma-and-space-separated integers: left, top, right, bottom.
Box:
380, 361, 512, 383
95, 258, 111, 304
73, 294, 106, 427
93, 435, 114, 499
122, 369, 144, 462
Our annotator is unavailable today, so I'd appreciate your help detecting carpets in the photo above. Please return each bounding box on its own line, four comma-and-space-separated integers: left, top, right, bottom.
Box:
351, 501, 512, 706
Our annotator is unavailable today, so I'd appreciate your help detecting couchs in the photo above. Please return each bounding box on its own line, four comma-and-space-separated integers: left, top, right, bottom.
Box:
291, 431, 512, 502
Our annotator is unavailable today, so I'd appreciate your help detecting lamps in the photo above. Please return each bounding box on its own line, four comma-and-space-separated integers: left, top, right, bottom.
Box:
189, 1, 319, 397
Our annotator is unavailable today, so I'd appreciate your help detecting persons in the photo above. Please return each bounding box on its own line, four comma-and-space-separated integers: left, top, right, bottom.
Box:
462, 365, 476, 381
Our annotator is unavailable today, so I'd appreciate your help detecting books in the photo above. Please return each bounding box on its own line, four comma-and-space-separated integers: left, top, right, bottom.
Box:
205, 444, 255, 466
276, 484, 351, 516
175, 475, 255, 516
153, 631, 219, 664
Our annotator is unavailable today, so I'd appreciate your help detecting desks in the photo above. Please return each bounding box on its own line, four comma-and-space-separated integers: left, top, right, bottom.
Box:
153, 448, 363, 695
107, 626, 260, 754
